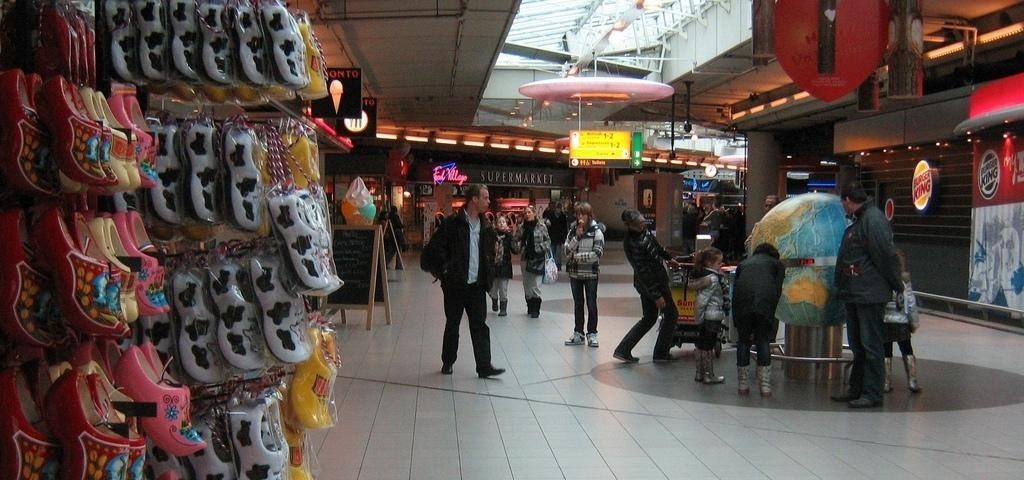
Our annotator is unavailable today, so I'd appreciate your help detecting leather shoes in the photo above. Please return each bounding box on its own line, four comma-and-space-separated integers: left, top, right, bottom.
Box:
477, 366, 506, 380
830, 387, 857, 402
438, 360, 455, 375
849, 393, 884, 409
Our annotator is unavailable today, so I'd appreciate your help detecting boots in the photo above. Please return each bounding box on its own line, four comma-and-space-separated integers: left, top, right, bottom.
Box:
703, 350, 725, 384
735, 364, 751, 393
693, 348, 705, 381
882, 357, 896, 392
489, 296, 543, 319
756, 365, 774, 396
902, 355, 923, 392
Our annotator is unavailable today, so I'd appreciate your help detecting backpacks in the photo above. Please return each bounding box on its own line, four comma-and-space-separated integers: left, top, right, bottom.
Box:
418, 212, 455, 274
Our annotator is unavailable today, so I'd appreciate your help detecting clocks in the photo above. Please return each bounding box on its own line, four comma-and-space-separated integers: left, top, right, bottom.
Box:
703, 137, 719, 178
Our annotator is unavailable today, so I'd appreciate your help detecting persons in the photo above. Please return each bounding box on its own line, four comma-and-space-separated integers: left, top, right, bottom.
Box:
513, 204, 552, 318
732, 242, 785, 397
564, 202, 606, 347
543, 200, 576, 272
682, 203, 747, 255
419, 182, 505, 378
687, 247, 732, 385
613, 207, 681, 363
486, 215, 520, 316
830, 181, 904, 408
764, 194, 779, 213
882, 248, 923, 393
378, 210, 390, 240
386, 205, 405, 270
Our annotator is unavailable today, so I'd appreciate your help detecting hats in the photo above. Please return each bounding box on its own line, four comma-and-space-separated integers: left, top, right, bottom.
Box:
621, 207, 643, 224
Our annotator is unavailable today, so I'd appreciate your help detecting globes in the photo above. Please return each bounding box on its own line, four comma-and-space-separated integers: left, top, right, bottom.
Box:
744, 192, 851, 327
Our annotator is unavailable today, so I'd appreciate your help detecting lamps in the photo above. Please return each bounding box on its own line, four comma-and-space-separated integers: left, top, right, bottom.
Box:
514, 48, 677, 105
609, 0, 708, 53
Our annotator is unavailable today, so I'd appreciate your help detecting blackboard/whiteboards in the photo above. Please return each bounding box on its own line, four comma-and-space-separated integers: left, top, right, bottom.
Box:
321, 225, 380, 310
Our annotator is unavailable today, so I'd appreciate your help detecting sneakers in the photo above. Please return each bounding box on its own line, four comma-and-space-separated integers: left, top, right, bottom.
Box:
587, 333, 599, 347
652, 347, 676, 364
566, 332, 585, 347
612, 348, 640, 363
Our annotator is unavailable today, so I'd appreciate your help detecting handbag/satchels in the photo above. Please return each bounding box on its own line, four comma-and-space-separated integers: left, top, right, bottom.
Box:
543, 257, 558, 285
736, 319, 779, 344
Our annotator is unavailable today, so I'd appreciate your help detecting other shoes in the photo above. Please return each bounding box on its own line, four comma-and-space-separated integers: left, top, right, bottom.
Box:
0, 0, 342, 480
